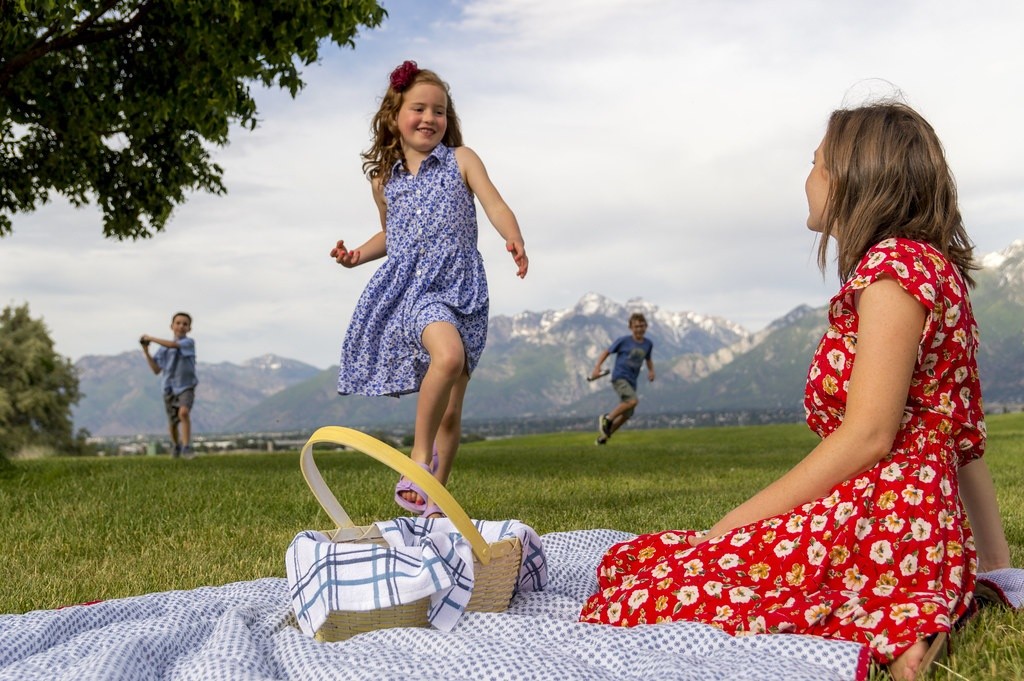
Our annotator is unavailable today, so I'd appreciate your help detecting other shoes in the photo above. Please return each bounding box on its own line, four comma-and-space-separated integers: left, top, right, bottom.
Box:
180, 445, 192, 455
172, 444, 181, 457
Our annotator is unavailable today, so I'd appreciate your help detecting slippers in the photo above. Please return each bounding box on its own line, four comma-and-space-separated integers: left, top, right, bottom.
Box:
886, 632, 950, 681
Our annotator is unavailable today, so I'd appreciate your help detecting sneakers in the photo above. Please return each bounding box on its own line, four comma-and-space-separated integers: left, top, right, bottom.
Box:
595, 436, 608, 447
599, 413, 612, 437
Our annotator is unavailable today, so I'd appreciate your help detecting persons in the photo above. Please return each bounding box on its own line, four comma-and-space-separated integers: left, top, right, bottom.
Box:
331, 62, 529, 519
579, 100, 1010, 681
140, 313, 198, 459
592, 313, 656, 445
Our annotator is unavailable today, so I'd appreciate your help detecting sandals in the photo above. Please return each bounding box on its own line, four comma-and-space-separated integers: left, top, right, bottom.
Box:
417, 504, 444, 519
396, 463, 431, 512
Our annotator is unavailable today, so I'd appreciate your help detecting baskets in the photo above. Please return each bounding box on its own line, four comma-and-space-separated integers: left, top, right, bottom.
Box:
286, 426, 522, 641
